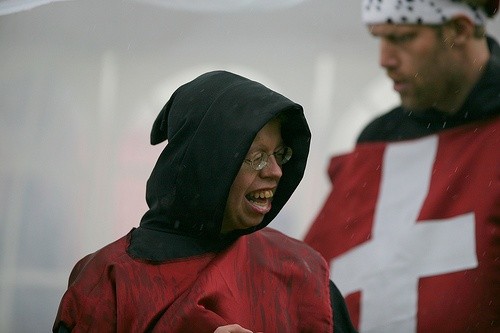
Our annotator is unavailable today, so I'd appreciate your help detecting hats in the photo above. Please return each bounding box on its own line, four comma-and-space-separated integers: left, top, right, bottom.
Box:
362, 0, 485, 29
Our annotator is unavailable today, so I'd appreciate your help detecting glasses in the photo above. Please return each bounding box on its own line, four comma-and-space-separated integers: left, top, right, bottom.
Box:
243, 145, 293, 170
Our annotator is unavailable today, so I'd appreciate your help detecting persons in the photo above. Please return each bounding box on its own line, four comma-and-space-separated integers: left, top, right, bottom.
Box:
297, 0, 498, 333
52, 69, 337, 333
